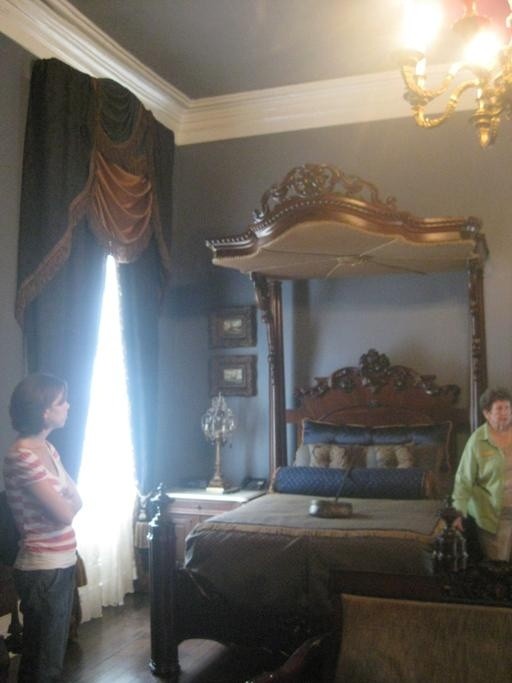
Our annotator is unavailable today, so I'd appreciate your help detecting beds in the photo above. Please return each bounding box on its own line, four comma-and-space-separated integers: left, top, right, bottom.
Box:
146, 349, 469, 677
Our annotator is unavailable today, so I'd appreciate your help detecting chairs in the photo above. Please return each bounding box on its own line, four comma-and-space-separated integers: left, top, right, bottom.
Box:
260, 627, 341, 683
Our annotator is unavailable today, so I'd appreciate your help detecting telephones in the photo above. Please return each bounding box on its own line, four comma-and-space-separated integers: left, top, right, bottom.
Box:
240, 476, 266, 490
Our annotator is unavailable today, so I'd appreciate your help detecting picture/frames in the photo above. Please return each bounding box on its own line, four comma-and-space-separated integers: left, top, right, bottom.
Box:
208, 354, 257, 397
207, 303, 257, 349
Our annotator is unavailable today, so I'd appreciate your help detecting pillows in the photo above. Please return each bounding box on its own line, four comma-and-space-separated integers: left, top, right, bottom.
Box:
336, 593, 511, 683
268, 417, 452, 500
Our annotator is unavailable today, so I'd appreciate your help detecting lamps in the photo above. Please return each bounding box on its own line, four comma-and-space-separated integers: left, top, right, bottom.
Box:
383, 2, 512, 147
199, 388, 238, 494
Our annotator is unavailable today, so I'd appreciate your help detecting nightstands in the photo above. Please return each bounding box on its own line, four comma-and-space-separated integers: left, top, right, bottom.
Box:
164, 487, 267, 561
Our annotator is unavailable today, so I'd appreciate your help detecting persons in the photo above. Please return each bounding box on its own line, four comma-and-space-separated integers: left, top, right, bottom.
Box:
2, 373, 84, 682
451, 386, 512, 563
